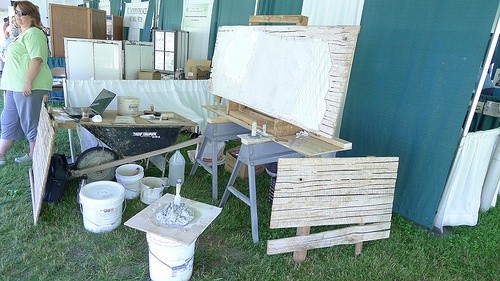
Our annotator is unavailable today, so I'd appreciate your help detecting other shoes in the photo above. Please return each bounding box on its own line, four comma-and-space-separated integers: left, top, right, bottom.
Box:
0, 156, 7, 165
15, 154, 33, 162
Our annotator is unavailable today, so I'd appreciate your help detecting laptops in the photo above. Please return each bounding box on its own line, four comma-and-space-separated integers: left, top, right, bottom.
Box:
63, 88, 116, 117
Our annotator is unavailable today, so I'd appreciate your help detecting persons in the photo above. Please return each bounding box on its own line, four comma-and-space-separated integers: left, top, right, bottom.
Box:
0, 0, 53, 164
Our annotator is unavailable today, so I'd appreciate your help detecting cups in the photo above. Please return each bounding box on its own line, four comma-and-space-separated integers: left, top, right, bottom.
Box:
82, 111, 90, 118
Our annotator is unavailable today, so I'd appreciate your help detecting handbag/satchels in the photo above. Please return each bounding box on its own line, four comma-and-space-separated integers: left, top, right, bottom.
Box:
43, 152, 70, 202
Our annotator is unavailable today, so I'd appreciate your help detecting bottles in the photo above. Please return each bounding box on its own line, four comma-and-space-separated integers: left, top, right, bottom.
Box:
175, 68, 184, 80
169, 150, 186, 187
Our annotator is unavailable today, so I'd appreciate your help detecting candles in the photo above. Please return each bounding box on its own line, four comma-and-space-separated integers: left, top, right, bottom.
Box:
252, 121, 257, 136
263, 124, 266, 134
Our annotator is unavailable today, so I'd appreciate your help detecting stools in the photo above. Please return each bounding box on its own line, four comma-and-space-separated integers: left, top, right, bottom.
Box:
218, 132, 305, 244
186, 116, 252, 200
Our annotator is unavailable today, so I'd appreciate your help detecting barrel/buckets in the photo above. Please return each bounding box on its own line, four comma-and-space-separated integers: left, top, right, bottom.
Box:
115, 164, 144, 200
139, 177, 170, 204
117, 96, 139, 117
79, 181, 126, 234
146, 232, 197, 281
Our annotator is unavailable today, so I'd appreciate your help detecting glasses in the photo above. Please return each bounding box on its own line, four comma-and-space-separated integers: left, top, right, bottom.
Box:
15, 9, 28, 16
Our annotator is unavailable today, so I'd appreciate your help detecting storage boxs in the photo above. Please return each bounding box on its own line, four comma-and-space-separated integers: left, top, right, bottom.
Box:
183, 58, 211, 80
137, 69, 161, 80
